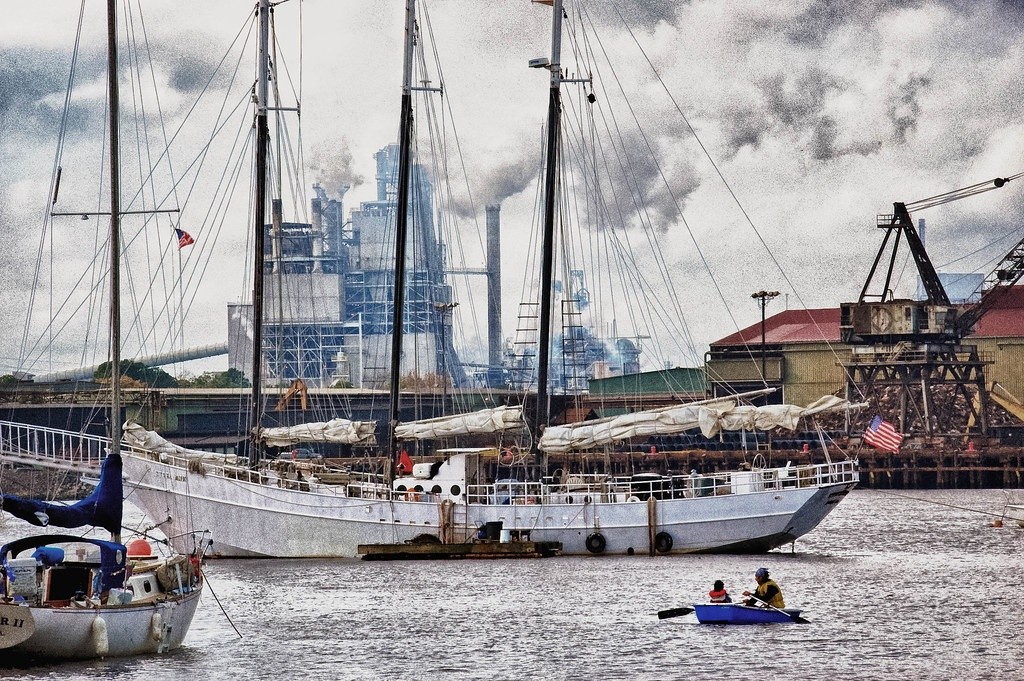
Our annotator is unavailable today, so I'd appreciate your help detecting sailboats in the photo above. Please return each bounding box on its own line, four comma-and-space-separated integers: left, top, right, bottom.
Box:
103, 2, 863, 559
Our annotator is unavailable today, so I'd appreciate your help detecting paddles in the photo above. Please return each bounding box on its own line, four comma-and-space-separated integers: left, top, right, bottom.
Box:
657, 601, 745, 620
748, 594, 811, 624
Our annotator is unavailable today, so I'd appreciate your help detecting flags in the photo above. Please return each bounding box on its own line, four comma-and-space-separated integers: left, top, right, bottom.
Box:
175, 228, 194, 250
863, 414, 904, 455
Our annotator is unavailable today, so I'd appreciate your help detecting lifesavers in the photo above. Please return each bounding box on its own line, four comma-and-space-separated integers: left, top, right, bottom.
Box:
654, 531, 674, 552
404, 488, 419, 502
584, 532, 607, 555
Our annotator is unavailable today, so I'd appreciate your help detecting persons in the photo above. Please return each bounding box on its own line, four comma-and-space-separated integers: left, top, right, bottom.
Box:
709, 580, 731, 603
743, 568, 785, 609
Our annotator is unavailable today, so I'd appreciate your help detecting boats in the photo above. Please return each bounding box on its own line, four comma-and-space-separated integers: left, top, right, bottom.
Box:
357, 521, 563, 559
691, 604, 800, 624
0, 533, 204, 664
1, 168, 1024, 489
